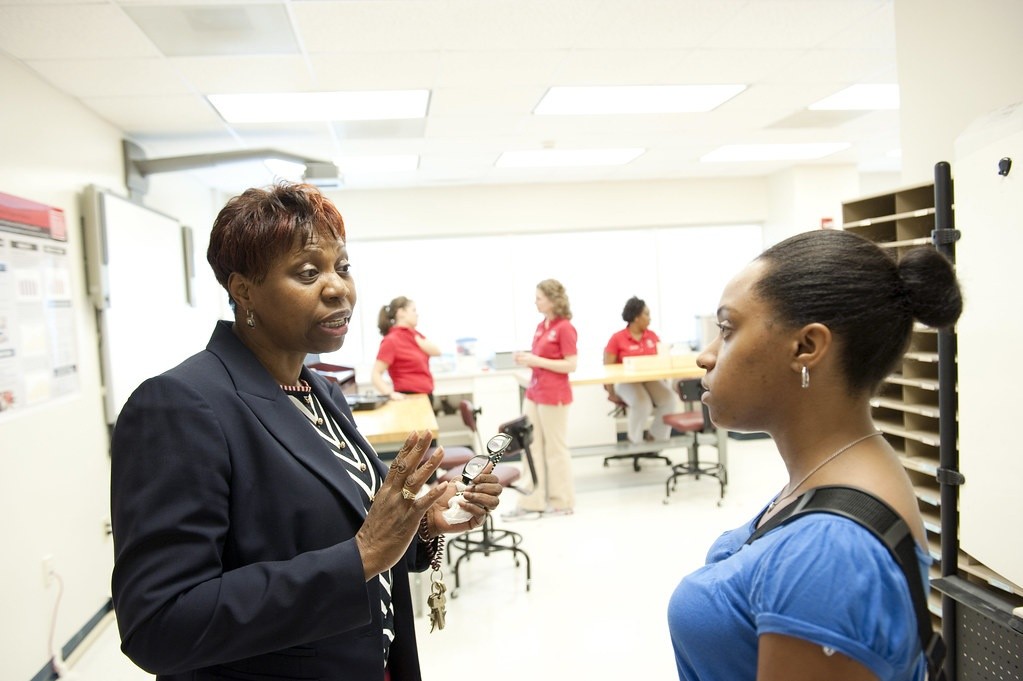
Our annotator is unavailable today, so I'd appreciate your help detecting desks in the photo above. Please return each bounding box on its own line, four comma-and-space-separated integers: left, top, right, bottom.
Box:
351, 393, 440, 485
513, 352, 727, 483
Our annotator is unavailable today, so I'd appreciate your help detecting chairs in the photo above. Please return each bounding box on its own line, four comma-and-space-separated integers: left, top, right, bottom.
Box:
663, 378, 727, 498
438, 415, 537, 600
425, 400, 484, 469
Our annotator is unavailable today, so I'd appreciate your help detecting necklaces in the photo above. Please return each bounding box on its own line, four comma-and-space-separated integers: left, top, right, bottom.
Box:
768, 431, 884, 512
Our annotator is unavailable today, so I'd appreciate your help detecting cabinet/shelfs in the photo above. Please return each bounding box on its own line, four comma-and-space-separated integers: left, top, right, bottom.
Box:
842, 179, 1023, 636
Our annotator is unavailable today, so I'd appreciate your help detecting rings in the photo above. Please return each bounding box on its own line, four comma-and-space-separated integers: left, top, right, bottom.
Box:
401, 487, 417, 500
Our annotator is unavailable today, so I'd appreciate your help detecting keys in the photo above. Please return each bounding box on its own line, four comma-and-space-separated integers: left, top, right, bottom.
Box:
428, 583, 447, 630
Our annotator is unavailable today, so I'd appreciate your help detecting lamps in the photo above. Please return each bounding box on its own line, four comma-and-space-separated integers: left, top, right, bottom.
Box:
122, 138, 339, 190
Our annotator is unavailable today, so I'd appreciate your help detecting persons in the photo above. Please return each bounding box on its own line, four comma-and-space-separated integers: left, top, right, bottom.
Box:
373, 296, 441, 486
668, 230, 964, 681
111, 187, 501, 681
605, 297, 675, 456
501, 281, 578, 521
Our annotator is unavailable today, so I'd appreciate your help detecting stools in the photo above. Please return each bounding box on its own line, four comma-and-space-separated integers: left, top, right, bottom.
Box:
603, 396, 672, 472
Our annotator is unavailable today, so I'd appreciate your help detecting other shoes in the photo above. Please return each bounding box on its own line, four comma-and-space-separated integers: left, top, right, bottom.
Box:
544, 502, 573, 519
500, 504, 539, 518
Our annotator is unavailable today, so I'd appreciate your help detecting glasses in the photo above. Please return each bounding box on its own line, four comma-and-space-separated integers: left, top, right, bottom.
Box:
461, 433, 513, 486
